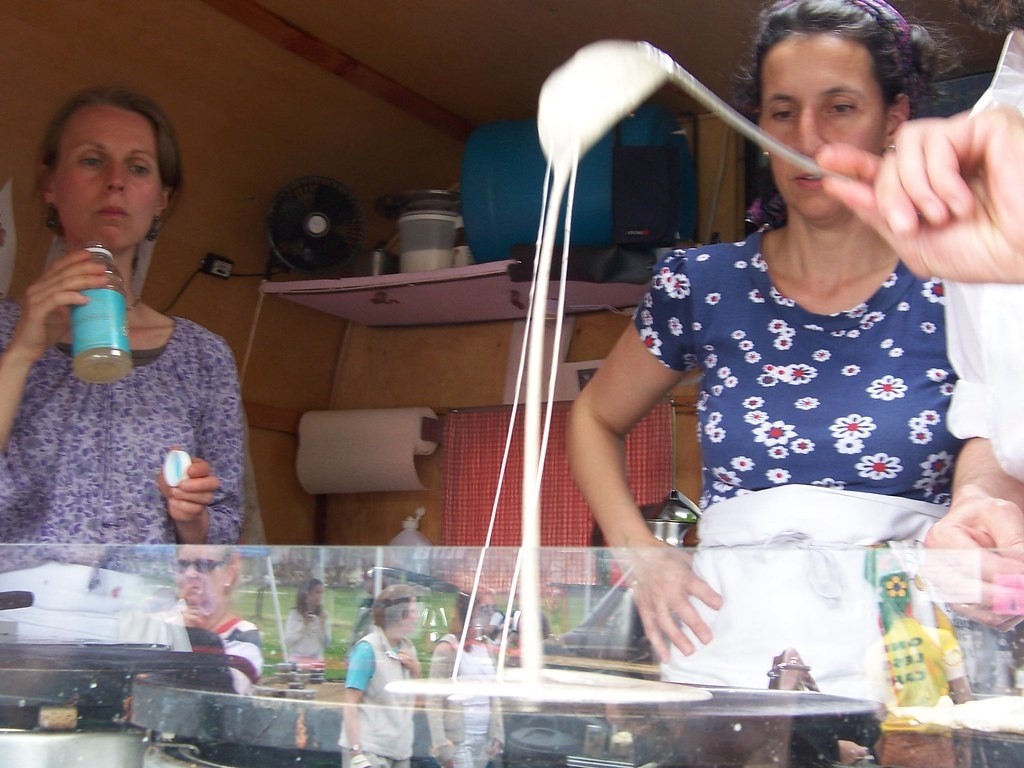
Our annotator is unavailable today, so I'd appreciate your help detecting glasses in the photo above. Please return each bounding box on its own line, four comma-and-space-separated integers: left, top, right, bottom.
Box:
476, 604, 497, 613
175, 559, 230, 573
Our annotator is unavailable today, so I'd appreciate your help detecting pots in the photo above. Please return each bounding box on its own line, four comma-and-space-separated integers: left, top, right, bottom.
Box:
0, 626, 888, 768
592, 490, 700, 659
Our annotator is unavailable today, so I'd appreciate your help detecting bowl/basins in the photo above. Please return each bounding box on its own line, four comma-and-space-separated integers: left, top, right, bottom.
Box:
360, 252, 398, 275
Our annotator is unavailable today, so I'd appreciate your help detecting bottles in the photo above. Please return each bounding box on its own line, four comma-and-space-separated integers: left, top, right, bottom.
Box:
71, 241, 133, 386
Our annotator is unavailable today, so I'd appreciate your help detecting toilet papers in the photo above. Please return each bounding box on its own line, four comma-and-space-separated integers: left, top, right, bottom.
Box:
295, 406, 440, 496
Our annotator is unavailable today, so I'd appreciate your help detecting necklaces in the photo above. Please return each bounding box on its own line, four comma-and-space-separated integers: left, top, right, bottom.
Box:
126, 300, 138, 311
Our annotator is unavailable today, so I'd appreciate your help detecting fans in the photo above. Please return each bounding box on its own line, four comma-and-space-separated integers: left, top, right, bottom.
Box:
266, 176, 366, 275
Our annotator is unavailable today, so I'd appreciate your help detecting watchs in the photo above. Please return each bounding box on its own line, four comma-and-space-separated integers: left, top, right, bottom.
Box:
348, 745, 360, 751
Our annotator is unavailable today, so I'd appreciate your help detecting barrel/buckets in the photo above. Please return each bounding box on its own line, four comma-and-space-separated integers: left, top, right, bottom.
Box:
400, 211, 461, 273
458, 105, 700, 263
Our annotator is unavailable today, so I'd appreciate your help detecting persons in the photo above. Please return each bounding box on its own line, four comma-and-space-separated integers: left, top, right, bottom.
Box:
567, 0, 968, 710
353, 570, 375, 635
284, 579, 331, 664
1, 84, 244, 580
338, 584, 420, 768
177, 545, 262, 693
427, 586, 505, 768
816, 0, 1023, 631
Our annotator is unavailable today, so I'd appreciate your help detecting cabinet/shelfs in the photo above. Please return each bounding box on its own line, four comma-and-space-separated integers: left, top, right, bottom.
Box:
240, 257, 698, 432
